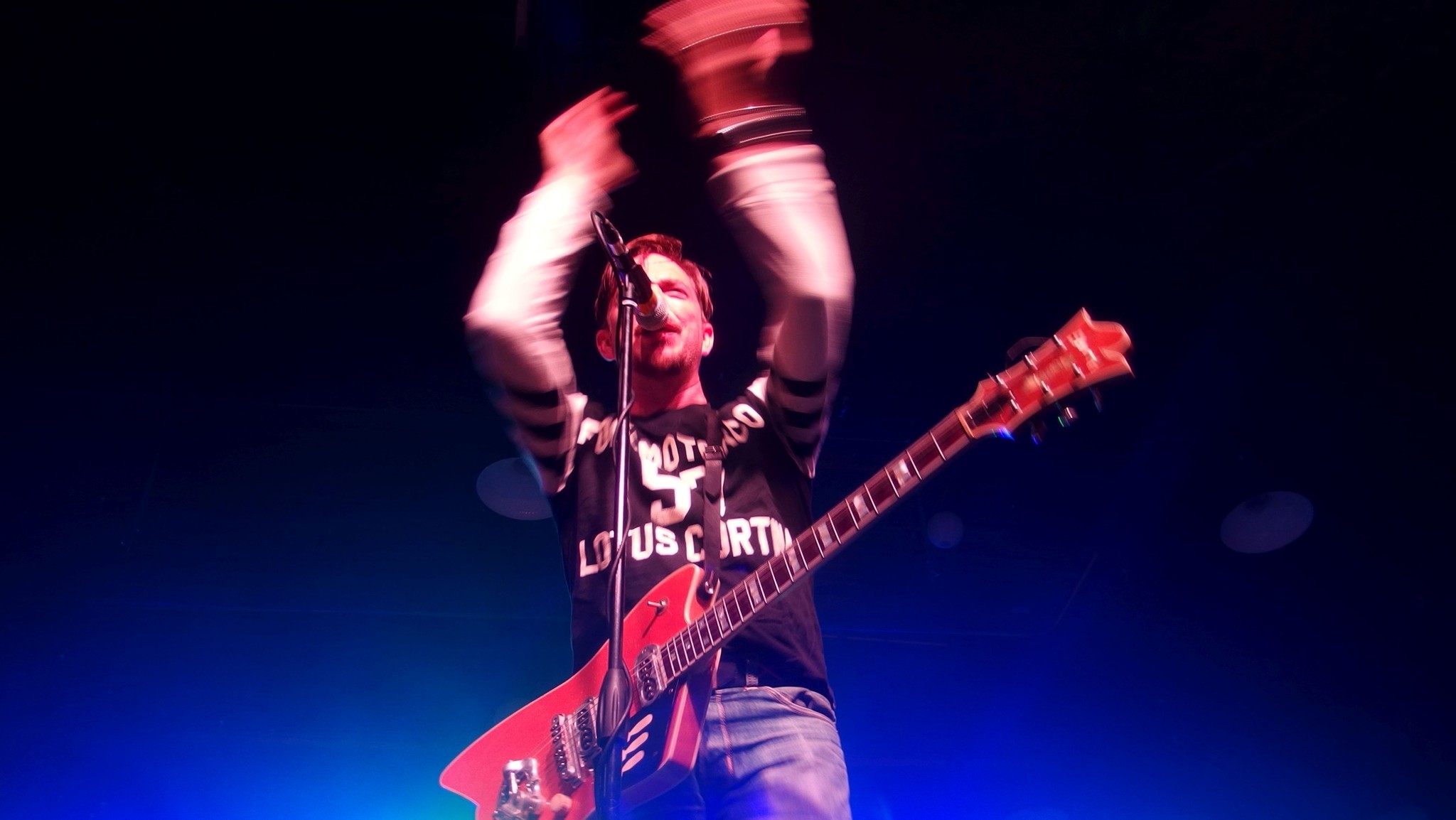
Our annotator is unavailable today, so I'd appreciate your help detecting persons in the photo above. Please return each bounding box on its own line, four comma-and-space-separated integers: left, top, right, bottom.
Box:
463, 0, 858, 820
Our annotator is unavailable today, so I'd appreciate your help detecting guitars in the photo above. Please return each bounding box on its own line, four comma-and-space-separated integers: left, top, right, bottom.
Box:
439, 304, 1133, 820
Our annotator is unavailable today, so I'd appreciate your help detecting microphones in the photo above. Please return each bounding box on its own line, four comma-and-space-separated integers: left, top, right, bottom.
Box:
601, 218, 669, 332
